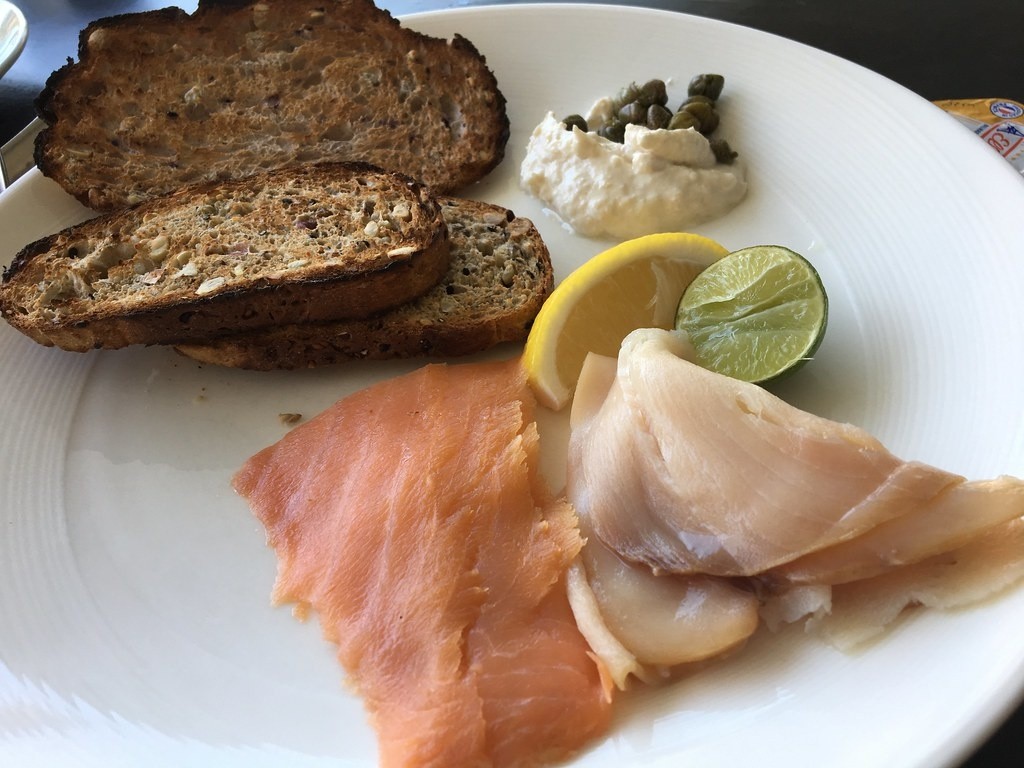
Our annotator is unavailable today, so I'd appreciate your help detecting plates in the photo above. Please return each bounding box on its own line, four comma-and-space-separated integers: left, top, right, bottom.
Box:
1, 2, 1024, 768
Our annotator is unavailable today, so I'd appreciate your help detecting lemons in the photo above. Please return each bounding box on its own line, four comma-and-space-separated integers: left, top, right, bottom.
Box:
676, 245, 829, 385
522, 232, 729, 409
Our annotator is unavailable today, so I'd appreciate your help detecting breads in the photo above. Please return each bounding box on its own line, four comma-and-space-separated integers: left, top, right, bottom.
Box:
171, 198, 555, 369
31, 0, 512, 210
0, 159, 449, 350
